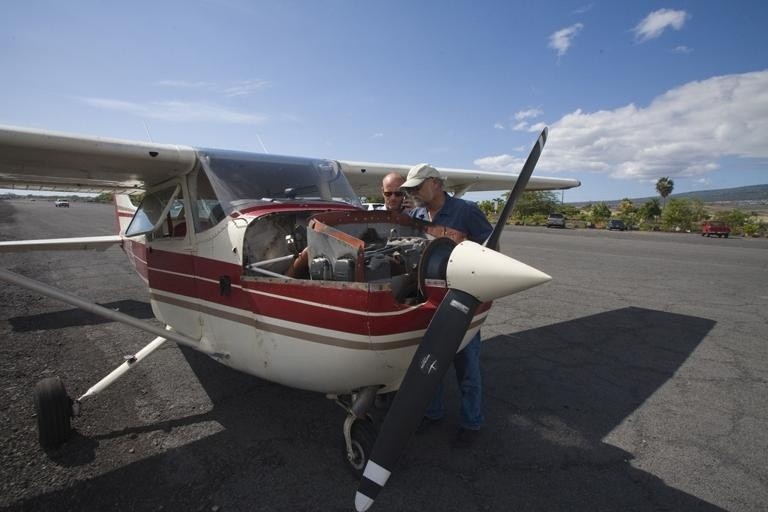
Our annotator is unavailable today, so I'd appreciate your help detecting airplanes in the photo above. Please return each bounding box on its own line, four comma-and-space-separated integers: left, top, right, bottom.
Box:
0, 126, 581, 512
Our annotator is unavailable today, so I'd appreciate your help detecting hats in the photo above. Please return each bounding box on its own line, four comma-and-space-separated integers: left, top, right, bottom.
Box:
400, 163, 441, 188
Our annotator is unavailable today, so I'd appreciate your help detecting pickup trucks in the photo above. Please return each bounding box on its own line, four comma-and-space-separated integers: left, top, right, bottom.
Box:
54, 198, 69, 208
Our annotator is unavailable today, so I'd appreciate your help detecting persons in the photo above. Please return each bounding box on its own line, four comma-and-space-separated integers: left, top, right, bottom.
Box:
402, 162, 500, 449
372, 172, 417, 215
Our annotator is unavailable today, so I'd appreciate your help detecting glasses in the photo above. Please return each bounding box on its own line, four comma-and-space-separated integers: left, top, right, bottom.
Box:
383, 190, 404, 197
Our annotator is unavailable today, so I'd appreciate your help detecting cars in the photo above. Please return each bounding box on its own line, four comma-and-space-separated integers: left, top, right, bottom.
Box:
700, 220, 731, 239
606, 219, 625, 231
545, 212, 566, 228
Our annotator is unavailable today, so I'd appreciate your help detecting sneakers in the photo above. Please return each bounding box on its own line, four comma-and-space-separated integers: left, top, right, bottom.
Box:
445, 423, 480, 449
